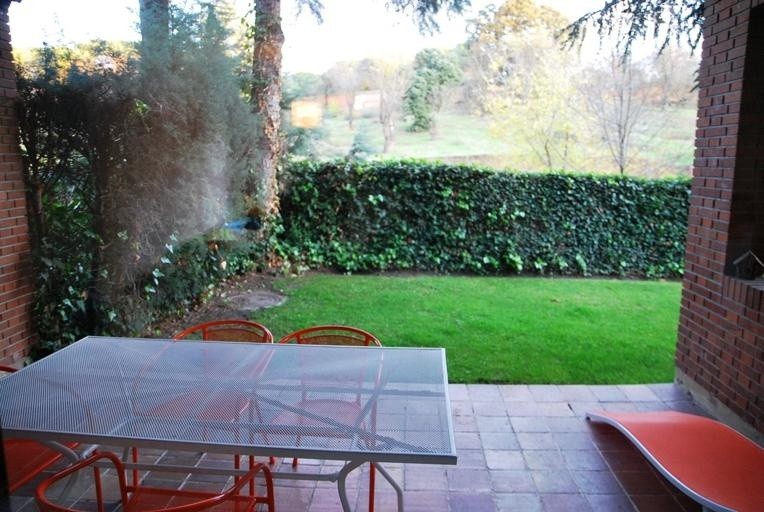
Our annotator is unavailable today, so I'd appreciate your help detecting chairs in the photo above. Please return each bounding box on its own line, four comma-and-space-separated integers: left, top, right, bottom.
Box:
33, 448, 277, 511
0, 358, 105, 511
254, 319, 385, 471
131, 320, 274, 493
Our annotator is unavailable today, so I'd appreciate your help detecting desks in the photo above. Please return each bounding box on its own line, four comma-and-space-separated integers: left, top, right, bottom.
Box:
0, 336, 458, 512
